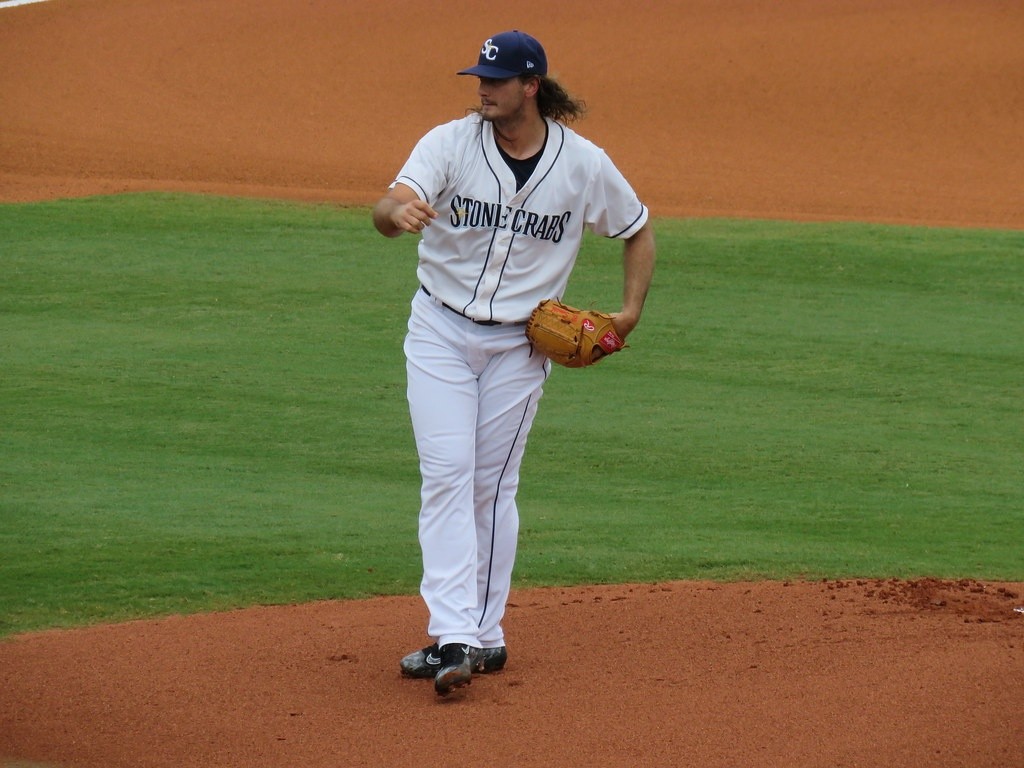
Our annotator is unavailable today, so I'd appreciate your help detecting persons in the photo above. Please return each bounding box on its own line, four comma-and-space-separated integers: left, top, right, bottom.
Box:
374, 30, 656, 697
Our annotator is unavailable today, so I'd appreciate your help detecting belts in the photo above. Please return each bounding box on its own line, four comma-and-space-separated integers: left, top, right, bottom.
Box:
421, 285, 530, 328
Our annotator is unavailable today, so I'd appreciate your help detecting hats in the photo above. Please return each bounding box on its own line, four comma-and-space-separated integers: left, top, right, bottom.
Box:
456, 30, 548, 78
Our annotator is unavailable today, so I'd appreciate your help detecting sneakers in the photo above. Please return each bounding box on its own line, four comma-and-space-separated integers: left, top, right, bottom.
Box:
400, 642, 506, 677
434, 643, 485, 696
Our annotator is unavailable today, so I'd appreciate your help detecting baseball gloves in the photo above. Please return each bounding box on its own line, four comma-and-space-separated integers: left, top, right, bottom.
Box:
524, 299, 627, 369
